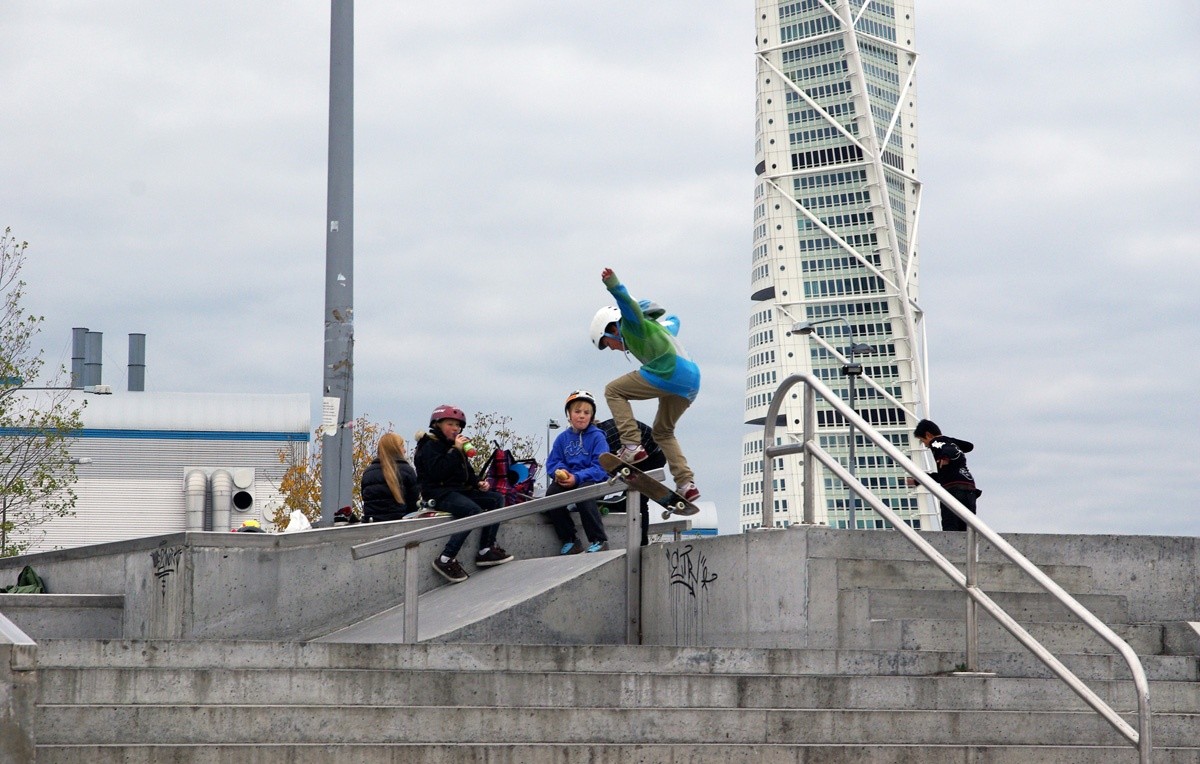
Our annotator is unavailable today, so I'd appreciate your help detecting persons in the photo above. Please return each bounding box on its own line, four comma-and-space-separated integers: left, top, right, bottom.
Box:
589, 267, 701, 503
414, 404, 514, 582
544, 389, 610, 555
592, 416, 667, 546
908, 419, 983, 532
361, 432, 420, 524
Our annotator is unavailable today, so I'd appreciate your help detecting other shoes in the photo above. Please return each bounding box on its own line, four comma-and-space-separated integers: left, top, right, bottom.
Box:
333, 507, 363, 527
560, 538, 583, 555
584, 540, 609, 553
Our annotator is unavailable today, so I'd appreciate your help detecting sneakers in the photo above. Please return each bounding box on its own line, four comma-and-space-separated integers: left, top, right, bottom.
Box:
432, 554, 470, 582
676, 482, 701, 502
475, 545, 515, 566
617, 445, 648, 464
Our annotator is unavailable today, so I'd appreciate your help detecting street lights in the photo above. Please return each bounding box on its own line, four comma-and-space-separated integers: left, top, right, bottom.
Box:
546, 417, 560, 493
786, 317, 864, 530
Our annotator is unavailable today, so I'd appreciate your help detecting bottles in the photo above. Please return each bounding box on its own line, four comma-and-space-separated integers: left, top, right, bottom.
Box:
496, 450, 507, 480
554, 469, 568, 481
454, 431, 477, 457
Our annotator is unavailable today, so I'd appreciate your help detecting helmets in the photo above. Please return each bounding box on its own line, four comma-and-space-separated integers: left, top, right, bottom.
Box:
565, 390, 597, 417
589, 305, 622, 350
429, 404, 466, 428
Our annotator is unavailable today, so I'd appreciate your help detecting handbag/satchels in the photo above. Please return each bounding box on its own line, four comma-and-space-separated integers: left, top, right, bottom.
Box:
285, 510, 311, 532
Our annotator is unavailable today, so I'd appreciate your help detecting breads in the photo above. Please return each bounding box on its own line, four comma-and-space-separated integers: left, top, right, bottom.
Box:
554, 469, 568, 479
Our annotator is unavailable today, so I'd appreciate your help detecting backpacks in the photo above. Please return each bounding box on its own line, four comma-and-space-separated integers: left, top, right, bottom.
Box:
7, 565, 47, 593
475, 440, 538, 507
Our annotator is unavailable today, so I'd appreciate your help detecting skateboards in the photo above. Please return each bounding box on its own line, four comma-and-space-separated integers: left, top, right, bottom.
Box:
598, 451, 700, 520
402, 499, 445, 519
516, 492, 627, 516
